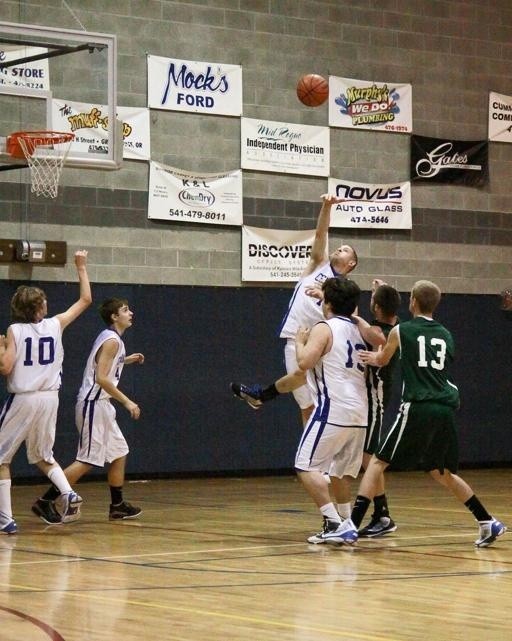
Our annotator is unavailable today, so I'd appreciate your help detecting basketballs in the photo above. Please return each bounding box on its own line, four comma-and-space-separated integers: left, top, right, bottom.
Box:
297, 74, 329, 107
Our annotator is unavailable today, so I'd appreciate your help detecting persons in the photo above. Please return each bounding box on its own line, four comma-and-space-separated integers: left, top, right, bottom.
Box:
1, 247, 91, 534
277, 193, 358, 485
292, 277, 381, 546
318, 281, 509, 546
30, 296, 147, 526
1, 527, 132, 641
227, 279, 405, 539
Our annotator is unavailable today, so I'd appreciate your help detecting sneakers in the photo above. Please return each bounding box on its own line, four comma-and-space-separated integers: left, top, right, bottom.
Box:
307, 515, 346, 544
473, 516, 507, 546
62, 491, 84, 523
0, 518, 17, 535
109, 500, 143, 520
229, 381, 264, 410
321, 518, 359, 545
31, 496, 63, 525
358, 513, 397, 537
